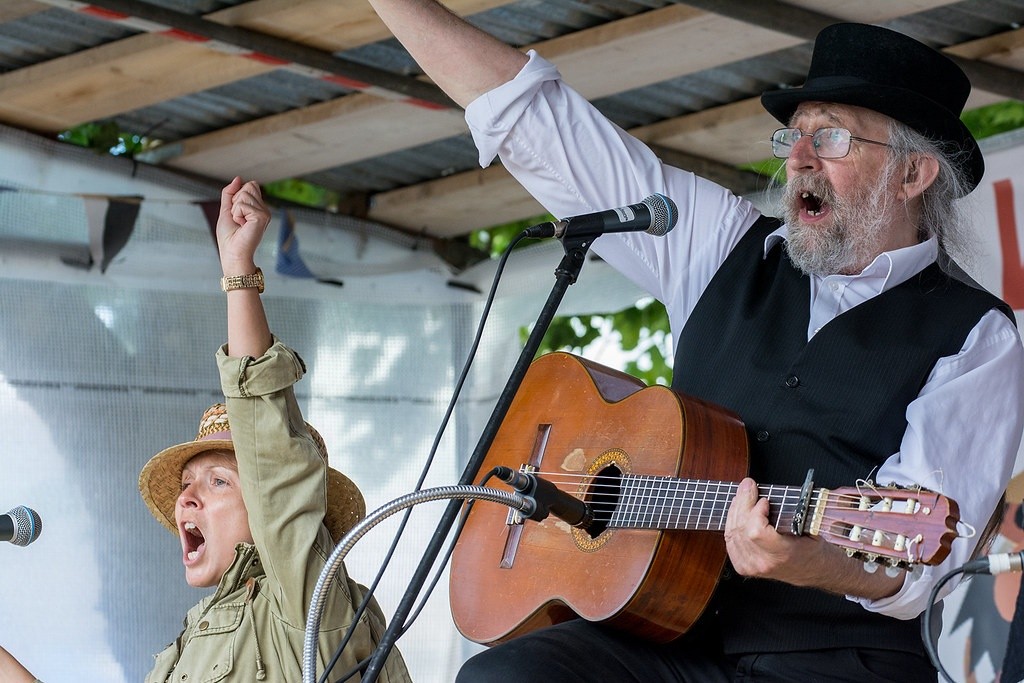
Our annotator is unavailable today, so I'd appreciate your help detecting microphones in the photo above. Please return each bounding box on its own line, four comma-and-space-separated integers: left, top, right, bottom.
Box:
0, 506, 42, 546
525, 194, 679, 237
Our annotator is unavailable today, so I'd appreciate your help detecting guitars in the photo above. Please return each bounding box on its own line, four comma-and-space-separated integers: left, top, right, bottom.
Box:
448, 352, 962, 648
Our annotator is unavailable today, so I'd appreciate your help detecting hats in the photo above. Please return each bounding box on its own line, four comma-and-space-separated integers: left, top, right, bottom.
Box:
139, 404, 366, 545
760, 21, 985, 199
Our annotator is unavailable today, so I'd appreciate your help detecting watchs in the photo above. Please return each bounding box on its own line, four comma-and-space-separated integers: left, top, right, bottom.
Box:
220, 267, 264, 294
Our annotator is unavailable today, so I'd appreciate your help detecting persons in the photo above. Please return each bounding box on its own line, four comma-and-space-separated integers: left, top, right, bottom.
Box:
366, 0, 1024, 683
0, 176, 412, 683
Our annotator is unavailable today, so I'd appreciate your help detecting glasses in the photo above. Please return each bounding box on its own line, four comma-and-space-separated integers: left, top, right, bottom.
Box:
771, 126, 920, 160
